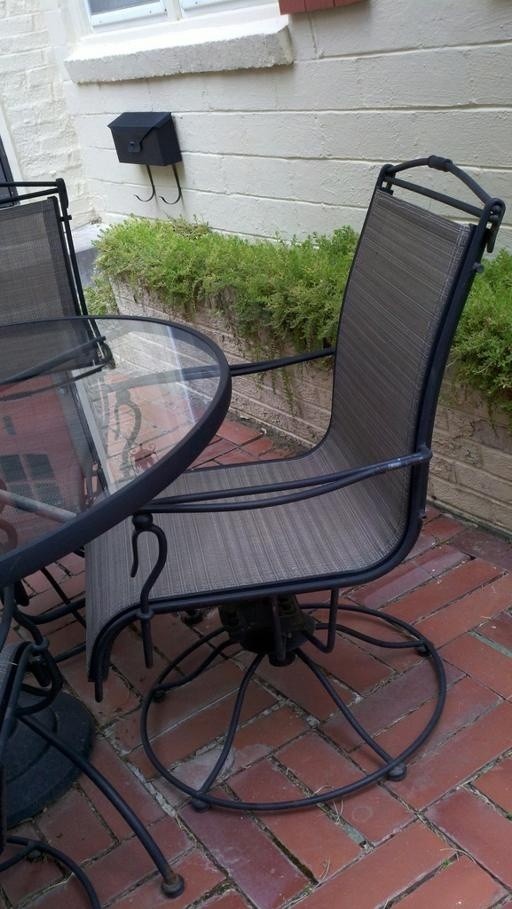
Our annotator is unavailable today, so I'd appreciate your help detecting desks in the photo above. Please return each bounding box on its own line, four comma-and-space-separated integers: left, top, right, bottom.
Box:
0, 318, 235, 829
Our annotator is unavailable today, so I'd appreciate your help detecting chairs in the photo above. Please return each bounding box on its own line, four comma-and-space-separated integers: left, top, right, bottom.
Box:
80, 156, 505, 813
0, 642, 186, 909
0, 177, 141, 480
